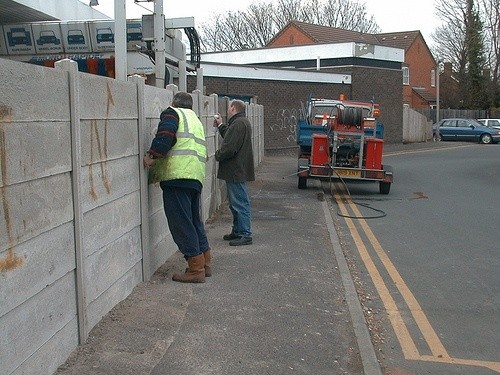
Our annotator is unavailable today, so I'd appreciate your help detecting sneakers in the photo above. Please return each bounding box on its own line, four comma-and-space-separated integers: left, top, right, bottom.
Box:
223, 234, 240, 240
230, 236, 252, 246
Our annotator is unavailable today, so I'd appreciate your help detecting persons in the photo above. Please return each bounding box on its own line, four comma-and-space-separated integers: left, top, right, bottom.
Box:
214, 100, 255, 246
144, 92, 212, 282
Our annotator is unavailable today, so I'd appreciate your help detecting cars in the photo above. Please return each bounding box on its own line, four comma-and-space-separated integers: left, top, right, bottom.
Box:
296, 95, 393, 195
478, 119, 500, 131
433, 118, 500, 144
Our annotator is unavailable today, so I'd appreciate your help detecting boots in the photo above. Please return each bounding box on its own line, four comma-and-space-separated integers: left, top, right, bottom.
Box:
185, 248, 211, 277
171, 253, 206, 283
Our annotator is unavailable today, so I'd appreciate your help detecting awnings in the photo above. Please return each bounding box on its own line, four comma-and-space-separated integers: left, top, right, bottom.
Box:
413, 88, 442, 102
